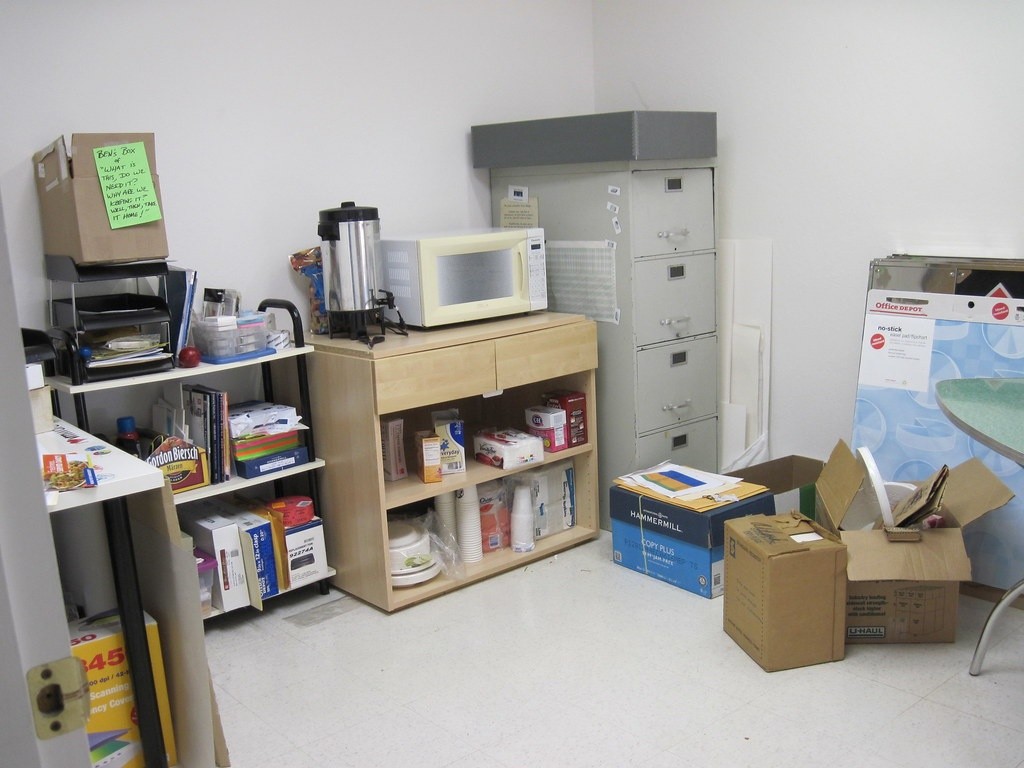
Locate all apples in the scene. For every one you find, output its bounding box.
[179,346,200,367]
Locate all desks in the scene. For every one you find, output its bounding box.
[935,376,1024,677]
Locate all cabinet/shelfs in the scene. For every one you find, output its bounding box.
[470,109,722,532]
[280,309,601,614]
[40,294,340,621]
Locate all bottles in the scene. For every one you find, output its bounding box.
[117,416,142,459]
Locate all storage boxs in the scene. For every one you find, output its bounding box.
[721,509,848,673]
[724,454,826,524]
[30,131,170,268]
[813,439,1016,644]
[609,467,775,600]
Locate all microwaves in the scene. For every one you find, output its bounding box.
[382,226,547,331]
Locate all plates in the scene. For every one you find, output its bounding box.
[50,460,88,489]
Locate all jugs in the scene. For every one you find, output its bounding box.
[317,200,395,340]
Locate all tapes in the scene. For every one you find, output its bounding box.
[266,329,291,350]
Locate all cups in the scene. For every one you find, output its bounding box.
[511,485,535,551]
[436,483,483,562]
